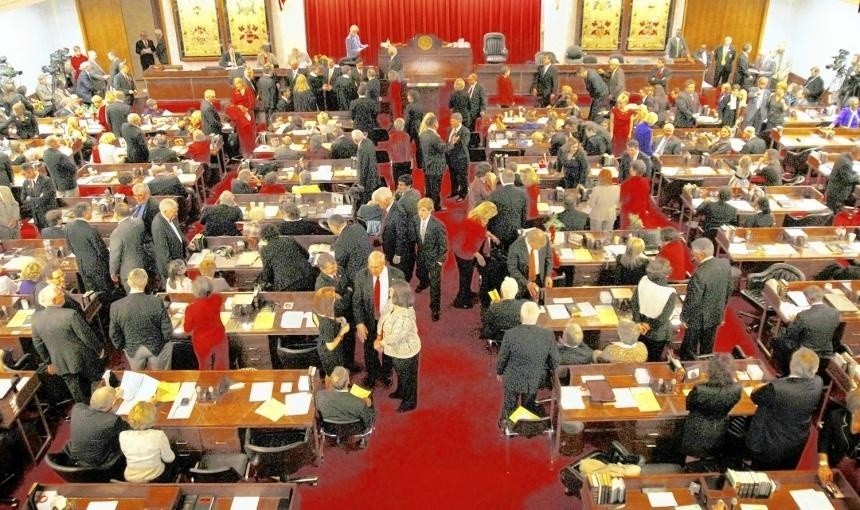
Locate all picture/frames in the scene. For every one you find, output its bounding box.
[575,0,626,56]
[170,0,227,62]
[219,0,276,61]
[623,0,676,56]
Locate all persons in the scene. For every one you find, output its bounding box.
[0,24,860,484]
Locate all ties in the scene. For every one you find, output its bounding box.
[528,249,536,281]
[372,276,380,321]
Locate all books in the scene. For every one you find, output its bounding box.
[586,473,626,506]
[725,468,776,498]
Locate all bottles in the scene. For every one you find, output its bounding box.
[655,377,679,395]
[194,384,219,403]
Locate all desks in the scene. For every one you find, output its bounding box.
[21,482,301,510]
[580,469,859,509]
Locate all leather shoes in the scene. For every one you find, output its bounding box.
[364,375,375,388]
[415,286,425,293]
[382,376,393,386]
[432,311,439,322]
[455,304,472,308]
[390,391,399,398]
[397,405,416,413]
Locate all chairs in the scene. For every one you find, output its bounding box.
[1,51,860,486]
[1,62,393,486]
[475,56,858,485]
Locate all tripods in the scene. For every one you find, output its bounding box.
[819,74,842,105]
